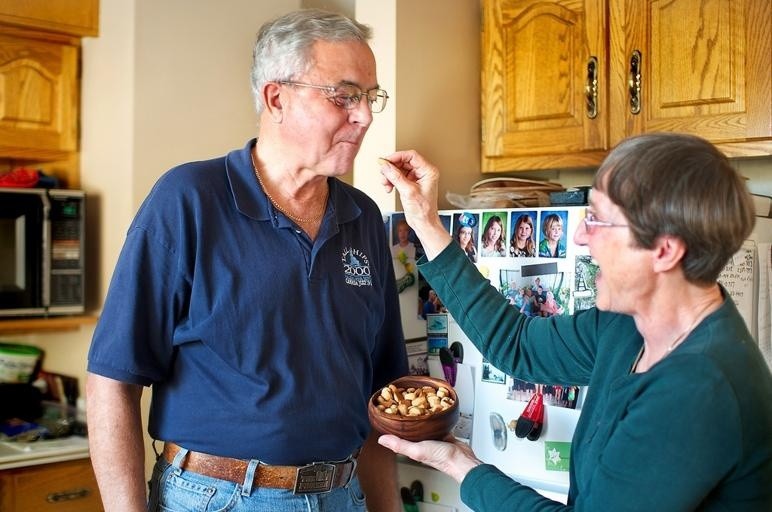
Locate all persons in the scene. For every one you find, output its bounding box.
[457,224,477,262]
[511,377,580,410]
[505,277,562,318]
[377,132,771,512]
[86,9,412,512]
[392,220,418,261]
[418,276,448,320]
[480,215,505,258]
[510,214,536,257]
[539,214,566,258]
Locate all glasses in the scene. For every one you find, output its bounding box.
[584,208,630,234]
[279,81,389,113]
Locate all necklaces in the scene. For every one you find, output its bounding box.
[250,153,329,222]
[630,295,721,374]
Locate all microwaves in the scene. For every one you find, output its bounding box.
[1,188,88,319]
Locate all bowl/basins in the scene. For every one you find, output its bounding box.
[367,376,460,442]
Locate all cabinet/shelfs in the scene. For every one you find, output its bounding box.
[0,32,81,152]
[1,457,105,510]
[477,2,771,172]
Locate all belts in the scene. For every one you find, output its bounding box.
[162,441,360,496]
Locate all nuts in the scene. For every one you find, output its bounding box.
[377,157,391,166]
[377,383,454,416]
